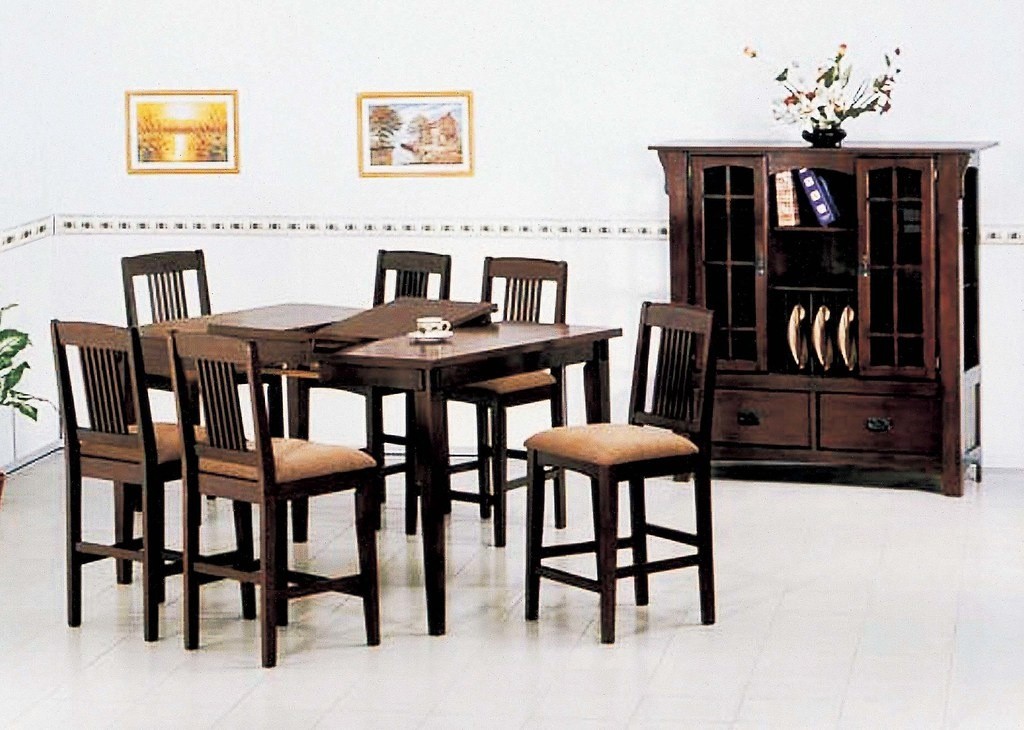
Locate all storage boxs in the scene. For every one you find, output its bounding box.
[775,171,802,227]
[798,169,839,225]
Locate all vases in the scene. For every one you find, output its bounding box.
[802,123,847,149]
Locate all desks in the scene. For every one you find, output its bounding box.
[127,301,624,636]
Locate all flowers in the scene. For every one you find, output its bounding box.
[742,42,901,134]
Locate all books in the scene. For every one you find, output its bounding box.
[774,168,840,227]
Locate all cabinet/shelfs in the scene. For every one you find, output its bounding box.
[646,140,998,498]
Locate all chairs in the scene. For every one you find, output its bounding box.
[307,247,451,528]
[166,328,381,667]
[122,249,283,501]
[525,302,716,644]
[50,320,255,643]
[406,258,569,547]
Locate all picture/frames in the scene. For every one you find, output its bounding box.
[357,92,474,179]
[125,91,240,175]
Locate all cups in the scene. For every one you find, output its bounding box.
[416,317,451,336]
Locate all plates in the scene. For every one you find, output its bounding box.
[407,331,453,343]
